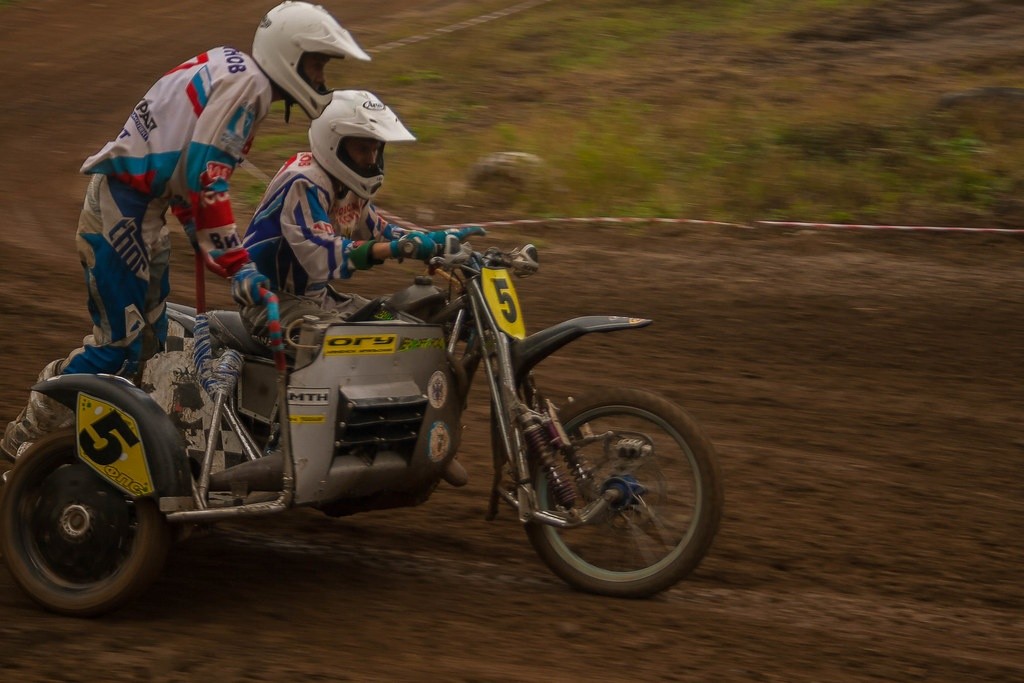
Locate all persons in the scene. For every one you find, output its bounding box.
[0,2,372,488]
[240,89,486,359]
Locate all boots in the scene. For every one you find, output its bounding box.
[0,358,79,465]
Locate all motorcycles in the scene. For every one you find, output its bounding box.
[1,236,724,614]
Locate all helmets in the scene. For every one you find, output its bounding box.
[304,88,417,200]
[252,0,372,122]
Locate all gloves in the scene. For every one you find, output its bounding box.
[424,224,487,259]
[230,262,271,309]
[391,231,438,265]
